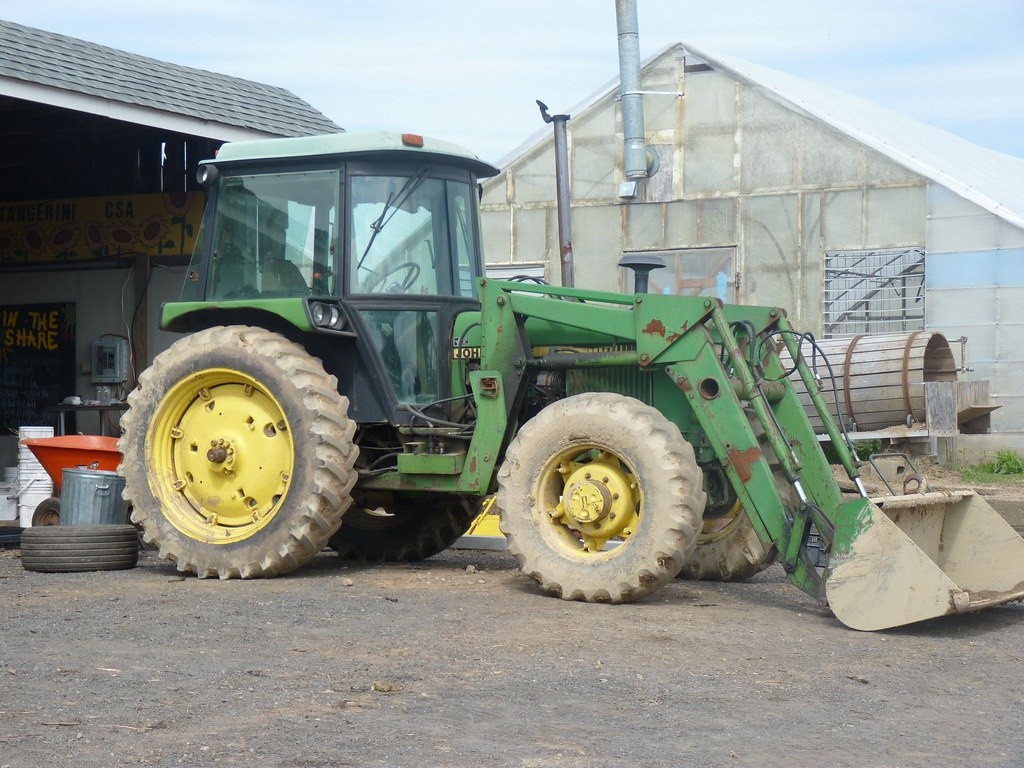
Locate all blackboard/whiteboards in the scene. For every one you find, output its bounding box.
[0,301,80,438]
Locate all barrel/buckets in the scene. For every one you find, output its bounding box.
[60,469,129,527]
[0,425,54,528]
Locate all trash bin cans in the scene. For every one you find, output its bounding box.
[61,467,130,528]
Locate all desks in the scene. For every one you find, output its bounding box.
[44,404,132,436]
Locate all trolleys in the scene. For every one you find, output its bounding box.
[20,433,123,528]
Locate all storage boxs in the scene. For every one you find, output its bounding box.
[0,494,20,520]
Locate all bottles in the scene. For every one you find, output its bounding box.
[96,386,112,406]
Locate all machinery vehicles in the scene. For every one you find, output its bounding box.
[115,1,1024,631]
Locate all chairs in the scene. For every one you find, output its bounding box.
[260,259,310,297]
